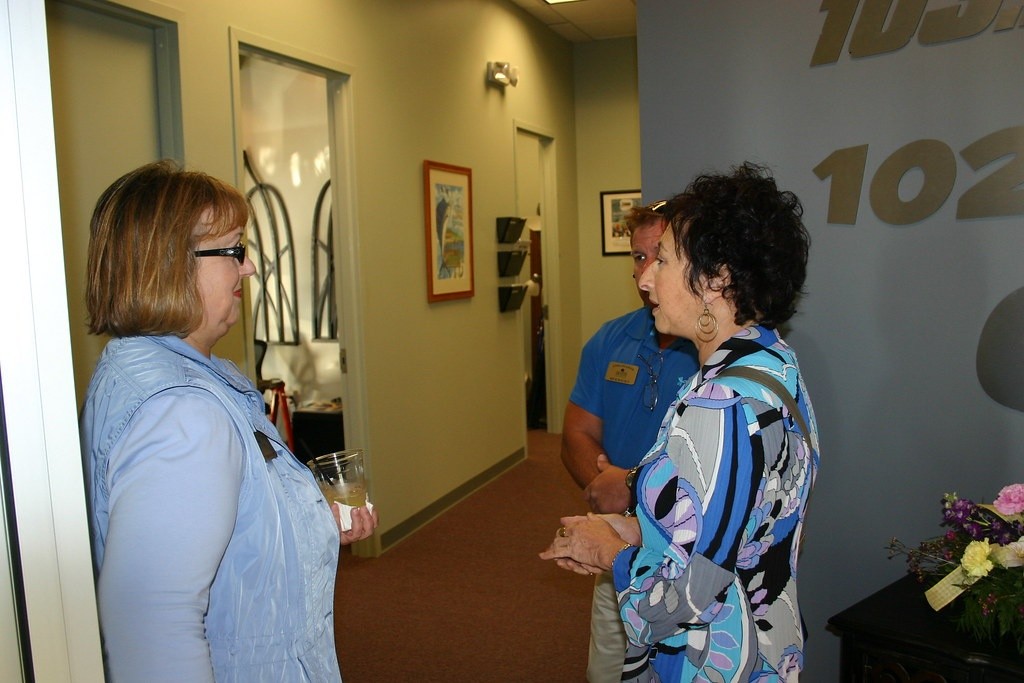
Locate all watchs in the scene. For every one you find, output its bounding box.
[625,466,638,491]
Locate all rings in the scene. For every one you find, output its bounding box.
[559,526,569,537]
[589,573,594,576]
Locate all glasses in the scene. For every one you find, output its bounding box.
[637,350,665,412]
[194,241,246,266]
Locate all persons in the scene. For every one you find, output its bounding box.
[77,158,380,683]
[537,160,822,683]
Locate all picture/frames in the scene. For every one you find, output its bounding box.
[600,189,643,256]
[422,160,474,301]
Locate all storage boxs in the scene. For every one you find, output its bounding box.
[497,250,527,277]
[497,217,526,244]
[498,285,528,313]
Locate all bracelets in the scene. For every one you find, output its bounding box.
[611,544,636,566]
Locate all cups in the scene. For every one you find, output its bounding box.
[307,449,366,510]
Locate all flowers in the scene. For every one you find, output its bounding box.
[884,483,1024,656]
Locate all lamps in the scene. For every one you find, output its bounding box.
[487,62,518,87]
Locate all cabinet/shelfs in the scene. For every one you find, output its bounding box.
[292,401,346,479]
[826,570,1024,683]
